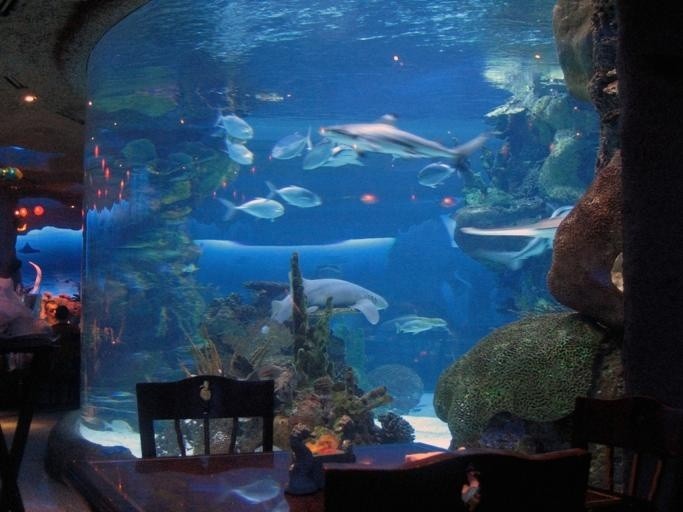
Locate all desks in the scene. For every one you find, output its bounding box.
[63,442,626,512]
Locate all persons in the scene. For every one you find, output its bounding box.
[51,306,80,369]
